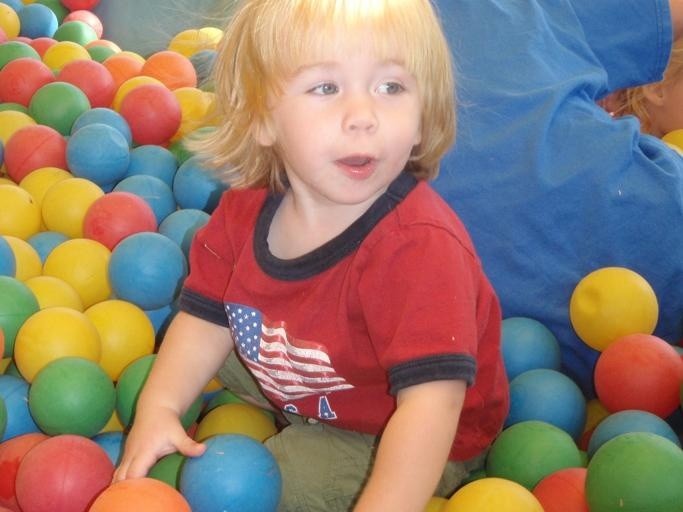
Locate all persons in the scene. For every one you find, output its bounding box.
[595,1,682,150]
[109,0,510,512]
[429,0,683,431]
